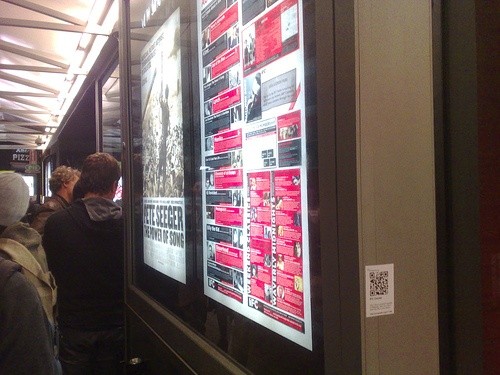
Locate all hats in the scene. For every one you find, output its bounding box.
[0,170,29,227]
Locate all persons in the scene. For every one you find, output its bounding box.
[0,165,80,375]
[154,84,170,199]
[44,153,126,375]
